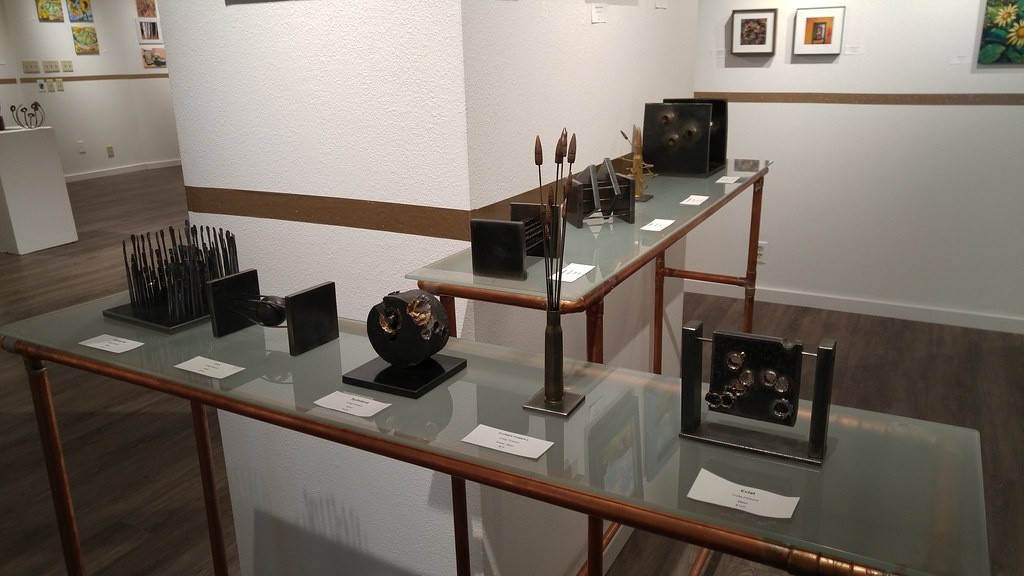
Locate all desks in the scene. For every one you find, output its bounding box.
[0,278,992,576]
[403,160,775,576]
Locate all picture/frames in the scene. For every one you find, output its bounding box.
[793,6,846,55]
[136,18,163,44]
[970,0,1024,73]
[731,8,778,54]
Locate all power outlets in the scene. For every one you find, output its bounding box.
[757,241,767,264]
[106,145,114,157]
[21,61,40,73]
[77,140,86,154]
[46,79,55,92]
[56,79,64,91]
[43,60,60,72]
[61,60,73,72]
